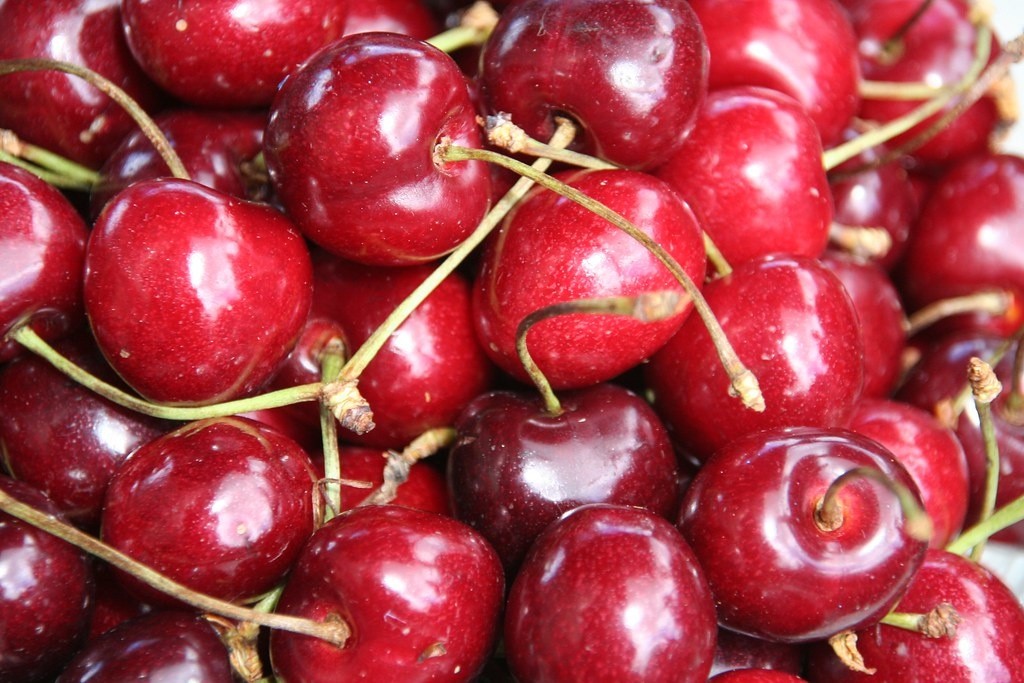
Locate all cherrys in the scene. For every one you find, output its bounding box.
[0,1,1024,683]
